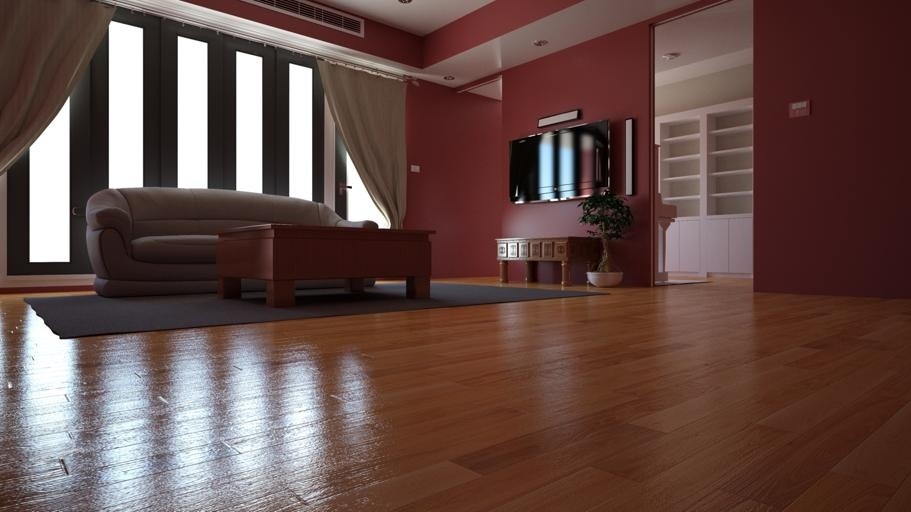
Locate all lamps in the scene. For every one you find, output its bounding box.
[538,109,580,128]
[625,119,635,196]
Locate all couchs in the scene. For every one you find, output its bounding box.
[85,187,378,298]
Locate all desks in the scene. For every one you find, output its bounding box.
[494,236,603,286]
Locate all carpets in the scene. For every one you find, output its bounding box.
[24,282,610,339]
[654,280,710,286]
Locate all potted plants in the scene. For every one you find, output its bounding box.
[576,187,636,288]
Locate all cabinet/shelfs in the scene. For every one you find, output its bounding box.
[655,97,753,278]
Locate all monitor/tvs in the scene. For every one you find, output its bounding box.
[509,120,610,205]
